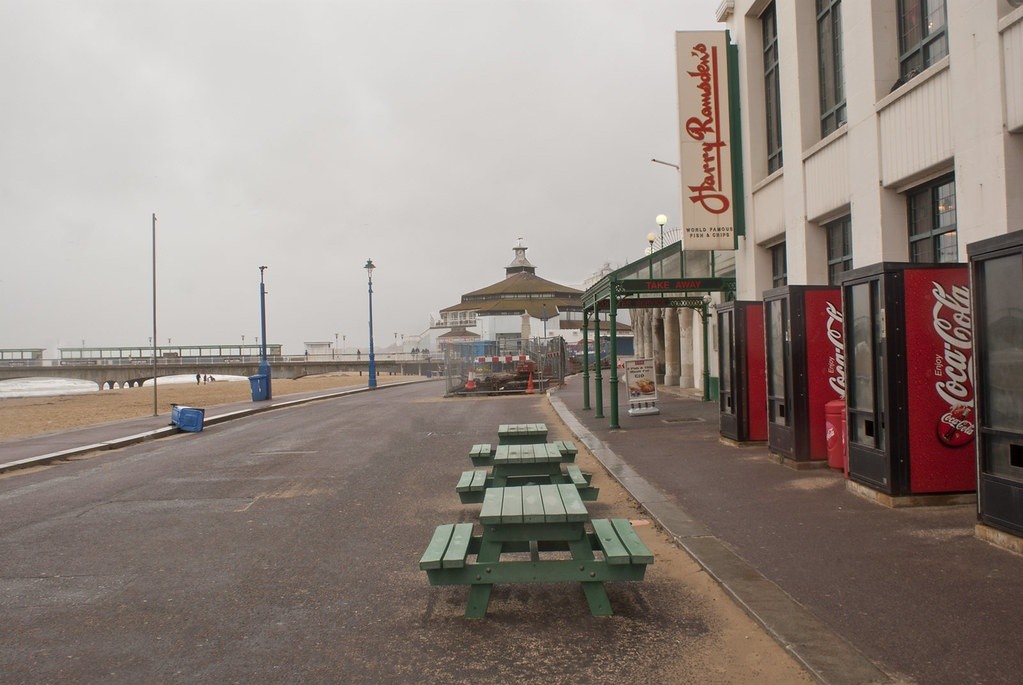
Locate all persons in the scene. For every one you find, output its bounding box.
[196,372,201,385]
[357,349,360,359]
[421,349,429,359]
[411,348,420,360]
[209,375,215,381]
[305,350,308,361]
[204,374,206,385]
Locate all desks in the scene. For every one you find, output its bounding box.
[463,423,614,620]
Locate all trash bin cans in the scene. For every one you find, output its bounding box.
[168,403,205,433]
[248,374,267,402]
[825,399,846,473]
[842,408,849,478]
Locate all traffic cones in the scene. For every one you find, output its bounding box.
[466,368,476,389]
[525,370,535,393]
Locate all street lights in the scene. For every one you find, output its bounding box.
[656,214,667,280]
[647,232,655,279]
[361,258,377,387]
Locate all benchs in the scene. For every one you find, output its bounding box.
[554,441,654,582]
[418,443,492,588]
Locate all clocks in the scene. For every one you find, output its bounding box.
[517,249,523,255]
